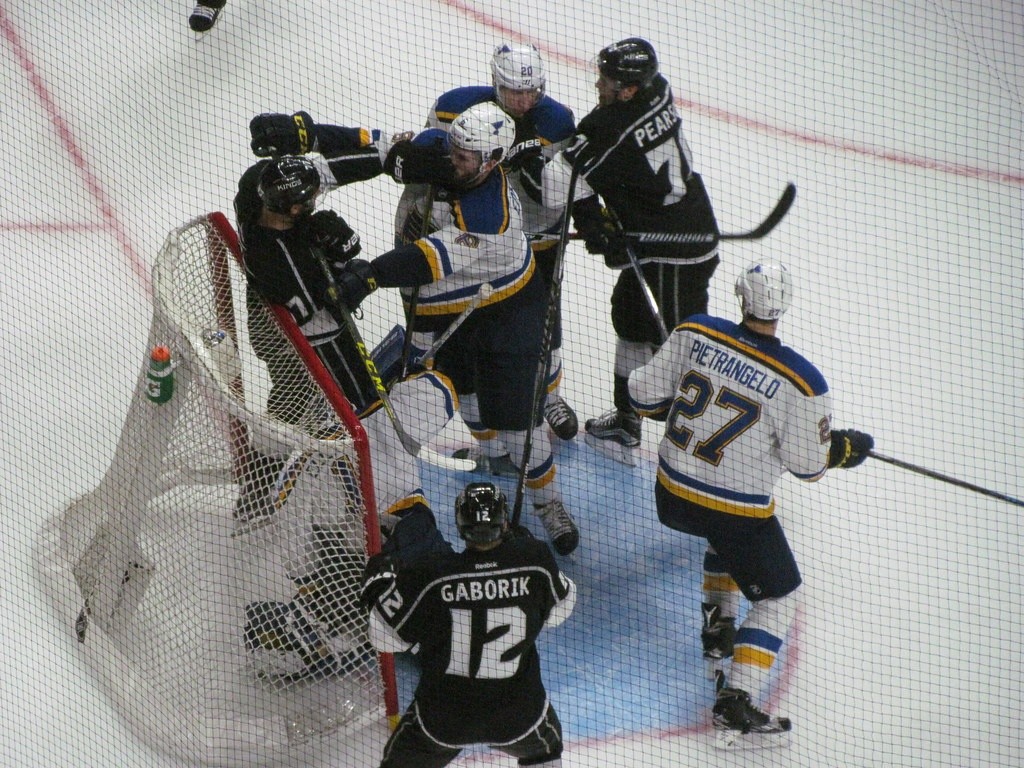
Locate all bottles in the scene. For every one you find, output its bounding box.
[143,346,174,404]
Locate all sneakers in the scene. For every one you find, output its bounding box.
[533,497,579,564]
[701,602,739,680]
[543,396,578,443]
[584,406,642,465]
[189,0,226,40]
[711,686,792,750]
[452,448,518,477]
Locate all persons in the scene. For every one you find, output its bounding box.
[395,42,601,447]
[251,101,580,563]
[630,256,875,750]
[189,0,227,42]
[516,38,722,465]
[233,140,378,518]
[244,370,458,653]
[366,483,577,768]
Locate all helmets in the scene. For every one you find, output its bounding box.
[454,482,508,542]
[735,259,793,319]
[257,154,320,214]
[489,41,545,90]
[598,37,658,86]
[448,101,515,165]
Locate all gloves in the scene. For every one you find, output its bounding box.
[828,428,874,470]
[249,110,316,157]
[362,553,399,599]
[325,260,375,313]
[584,223,615,255]
[382,139,457,185]
[309,209,362,263]
[573,194,604,255]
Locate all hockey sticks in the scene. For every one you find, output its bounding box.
[523,180,797,245]
[397,183,436,380]
[595,192,673,338]
[871,448,1023,508]
[511,165,579,528]
[413,294,480,365]
[312,242,479,474]
